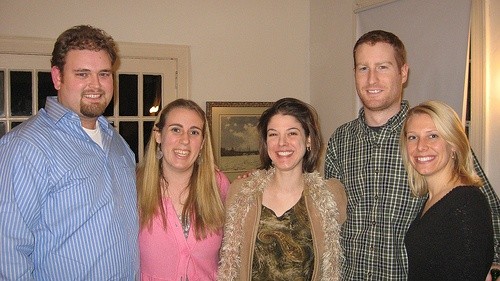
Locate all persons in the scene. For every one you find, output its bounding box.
[134,98,253,281]
[399,100,496,281]
[217,97,347,280]
[322,28,500,281]
[0,24,142,281]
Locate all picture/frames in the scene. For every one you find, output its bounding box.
[206,101,276,184]
